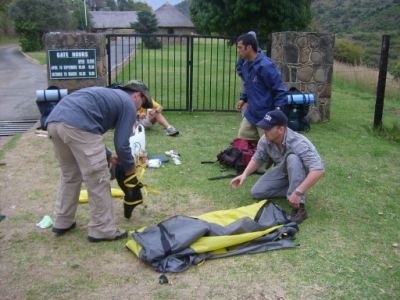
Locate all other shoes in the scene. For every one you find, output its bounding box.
[166,125,179,136]
[291,205,307,223]
[51,222,77,235]
[88,229,129,243]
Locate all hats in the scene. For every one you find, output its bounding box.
[255,110,289,130]
[114,79,154,109]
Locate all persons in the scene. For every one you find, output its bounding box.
[234,30,265,116]
[130,97,180,136]
[236,32,289,175]
[43,80,153,243]
[231,110,325,226]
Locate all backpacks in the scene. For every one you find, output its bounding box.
[286,87,311,133]
[201,137,258,181]
[35,85,68,130]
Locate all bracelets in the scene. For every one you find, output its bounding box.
[294,187,304,197]
